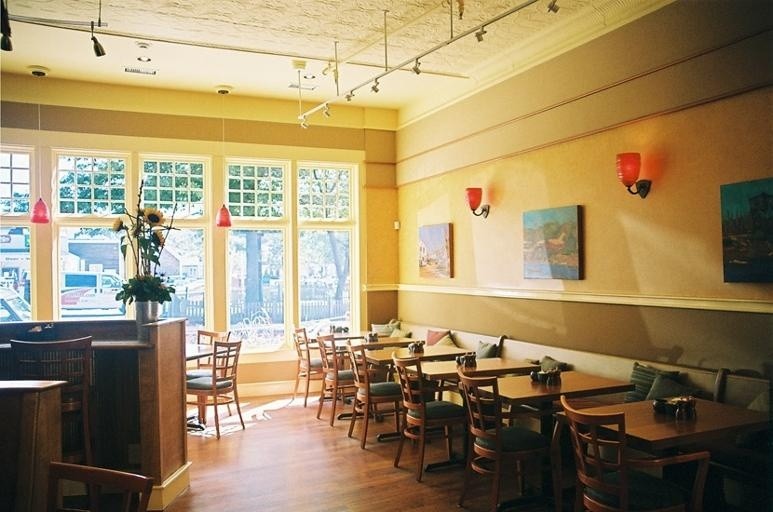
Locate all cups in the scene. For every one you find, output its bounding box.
[530,370,539,381]
[649,397,669,414]
[454,355,464,365]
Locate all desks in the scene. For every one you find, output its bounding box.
[313,329,391,339]
[355,344,476,366]
[184,339,234,360]
[330,338,426,351]
[404,357,543,406]
[476,368,637,438]
[555,396,770,512]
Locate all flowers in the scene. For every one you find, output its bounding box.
[104,175,186,302]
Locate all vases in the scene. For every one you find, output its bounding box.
[133,295,156,342]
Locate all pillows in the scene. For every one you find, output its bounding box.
[747,391,770,415]
[476,340,497,359]
[622,362,681,402]
[644,370,714,403]
[426,329,450,346]
[434,334,456,347]
[370,322,401,338]
[389,328,412,338]
[542,356,567,373]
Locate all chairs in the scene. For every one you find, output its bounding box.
[6,337,97,466]
[294,328,334,407]
[344,338,403,449]
[316,331,360,426]
[559,395,711,511]
[187,342,243,443]
[42,458,156,511]
[390,351,464,482]
[457,364,551,510]
[186,331,231,423]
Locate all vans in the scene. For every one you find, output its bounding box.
[61,272,126,314]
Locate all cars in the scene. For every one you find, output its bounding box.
[0,287,30,321]
[161,274,351,300]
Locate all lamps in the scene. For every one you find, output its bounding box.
[295,0,557,130]
[0,2,15,50]
[214,82,233,229]
[87,2,107,58]
[614,151,653,200]
[28,63,48,223]
[464,184,490,220]
